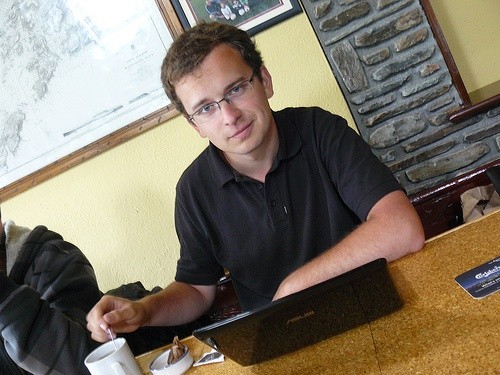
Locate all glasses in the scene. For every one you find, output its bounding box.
[187,71,255,123]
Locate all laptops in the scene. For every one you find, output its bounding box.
[193,258,403,366]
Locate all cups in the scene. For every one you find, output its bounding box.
[84,337,145,375]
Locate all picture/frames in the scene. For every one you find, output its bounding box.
[169,0,304,41]
[0,0,184,203]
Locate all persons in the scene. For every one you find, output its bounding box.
[203,0,251,21]
[0,209,101,375]
[84,23,427,345]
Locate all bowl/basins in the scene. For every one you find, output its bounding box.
[148,344,193,375]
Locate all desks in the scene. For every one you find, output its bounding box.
[132,211,499,375]
